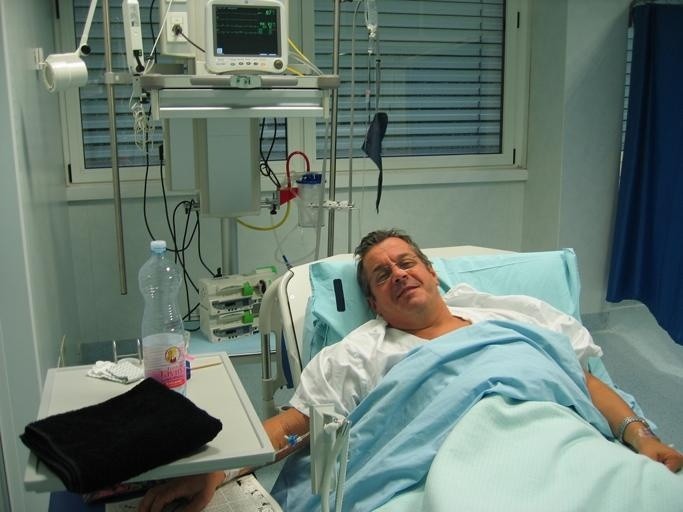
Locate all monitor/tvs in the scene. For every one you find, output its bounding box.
[209,0,287,75]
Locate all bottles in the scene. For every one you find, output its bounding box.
[137,238,187,399]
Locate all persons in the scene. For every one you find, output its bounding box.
[138,227,683,512]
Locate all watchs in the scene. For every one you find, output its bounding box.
[617,415,651,445]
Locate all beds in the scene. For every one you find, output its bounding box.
[258,244,683,512]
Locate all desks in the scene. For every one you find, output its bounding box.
[23,351,276,510]
